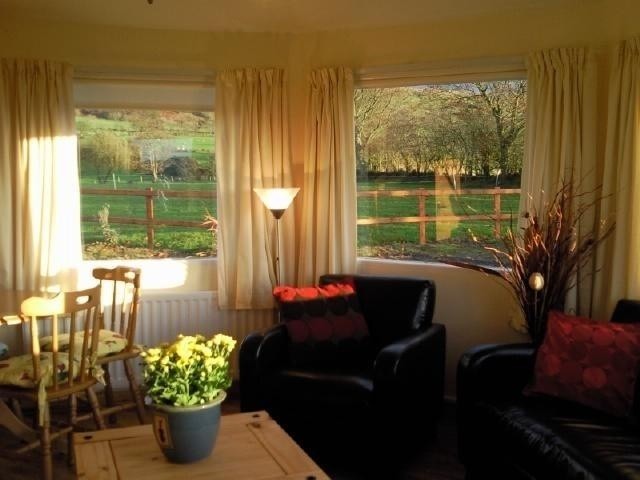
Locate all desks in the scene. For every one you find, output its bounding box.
[69,407,332,480]
[0,287,96,454]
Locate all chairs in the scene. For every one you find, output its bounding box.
[1,284,113,478]
[234,269,452,454]
[33,265,150,430]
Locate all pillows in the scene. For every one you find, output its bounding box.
[271,278,377,365]
[523,303,639,424]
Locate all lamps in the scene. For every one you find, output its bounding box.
[252,182,303,324]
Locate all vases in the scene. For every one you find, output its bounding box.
[137,387,228,465]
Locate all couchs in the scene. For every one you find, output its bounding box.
[451,296,639,479]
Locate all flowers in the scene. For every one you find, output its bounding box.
[129,327,245,411]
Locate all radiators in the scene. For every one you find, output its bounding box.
[19,287,280,396]
